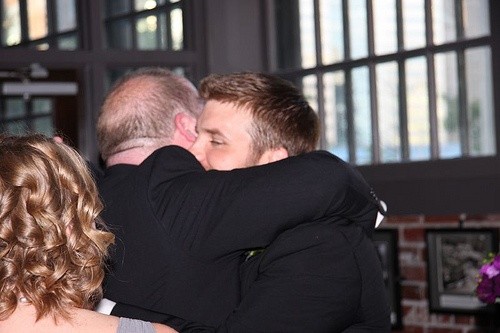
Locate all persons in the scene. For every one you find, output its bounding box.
[0,132,180,333]
[89,67,392,333]
